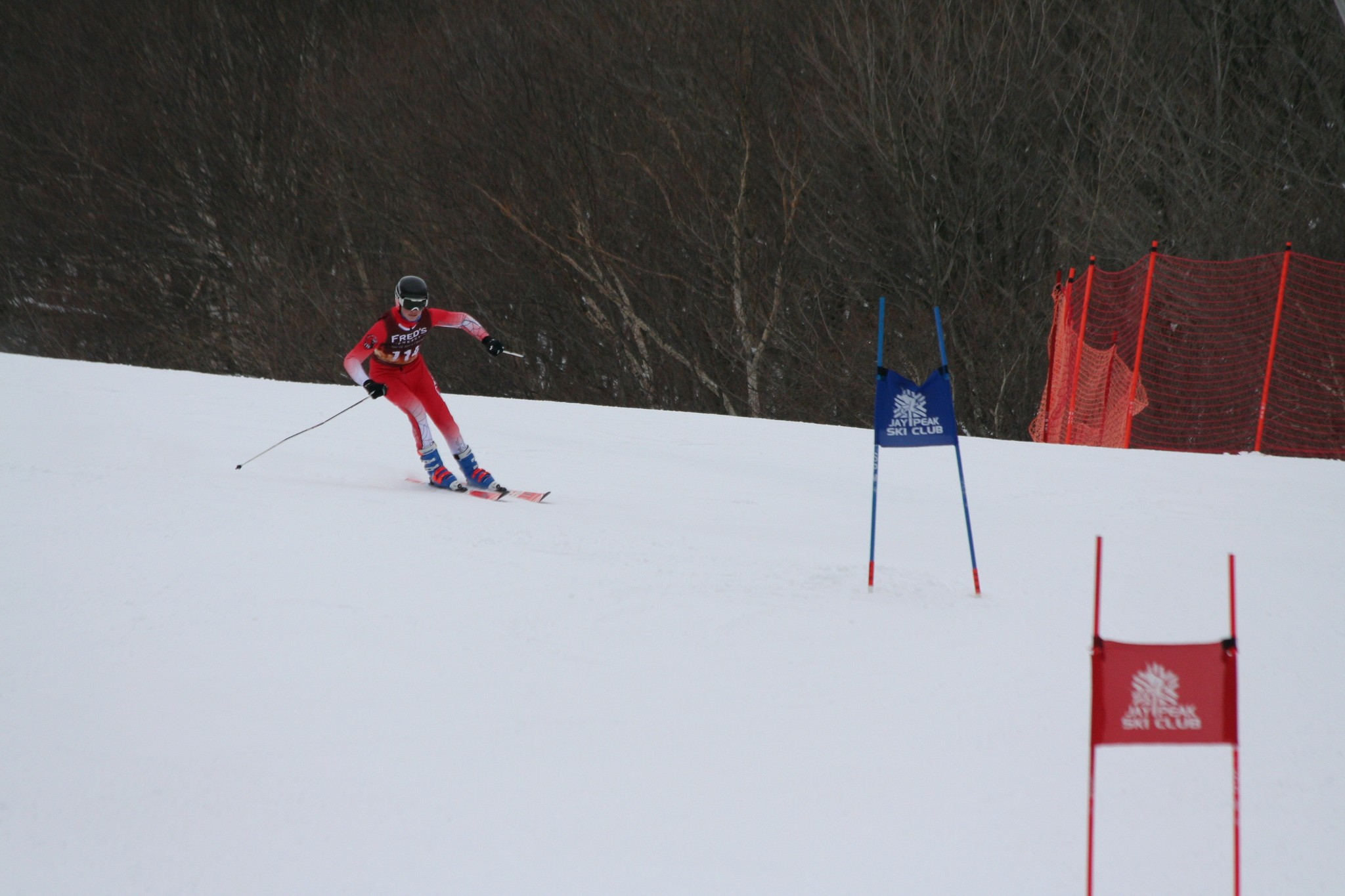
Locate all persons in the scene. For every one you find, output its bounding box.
[343,275,506,492]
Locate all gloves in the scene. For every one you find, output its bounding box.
[363,379,387,399]
[482,336,503,354]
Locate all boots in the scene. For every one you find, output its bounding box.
[454,445,498,489]
[417,442,459,488]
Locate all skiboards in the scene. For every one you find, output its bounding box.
[404,472,553,505]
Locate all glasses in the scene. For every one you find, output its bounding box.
[401,298,425,311]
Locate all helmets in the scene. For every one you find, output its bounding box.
[396,275,428,301]
